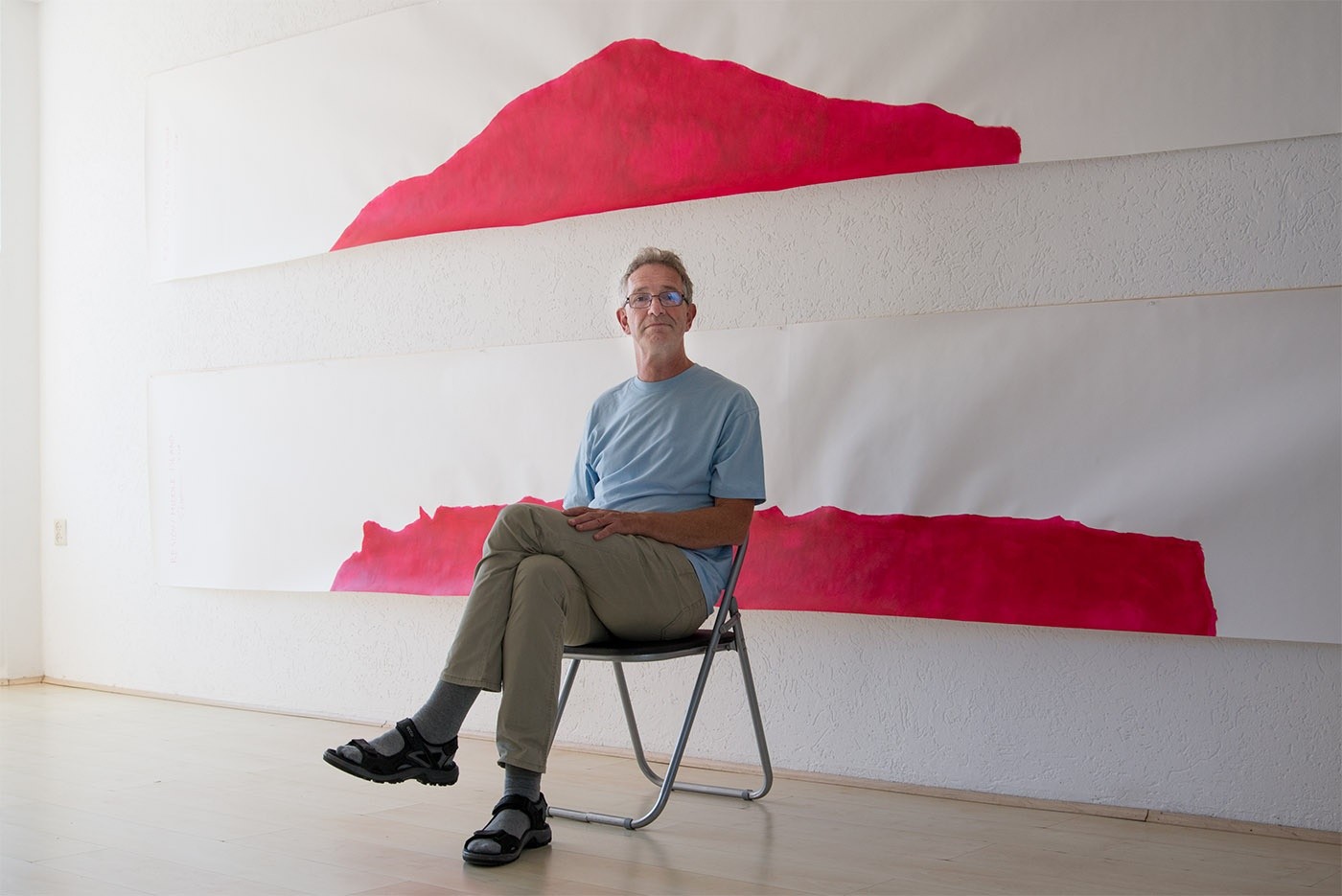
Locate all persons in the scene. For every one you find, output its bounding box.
[322,248,766,868]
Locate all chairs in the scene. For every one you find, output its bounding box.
[545,530,772,829]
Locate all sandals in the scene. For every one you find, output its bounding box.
[323,718,461,787]
[461,792,555,868]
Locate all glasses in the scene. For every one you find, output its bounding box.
[623,290,689,309]
